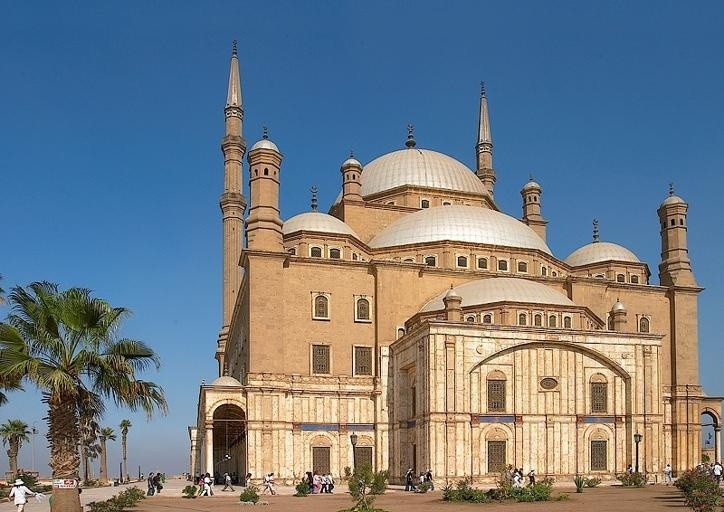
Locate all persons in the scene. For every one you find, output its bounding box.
[404,467,433,491]
[139,471,334,497]
[516,467,525,483]
[526,470,535,486]
[512,469,522,488]
[696,461,724,486]
[626,463,633,478]
[664,463,672,484]
[7,478,36,512]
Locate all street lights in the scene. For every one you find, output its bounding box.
[350,432,357,469]
[634,429,643,474]
